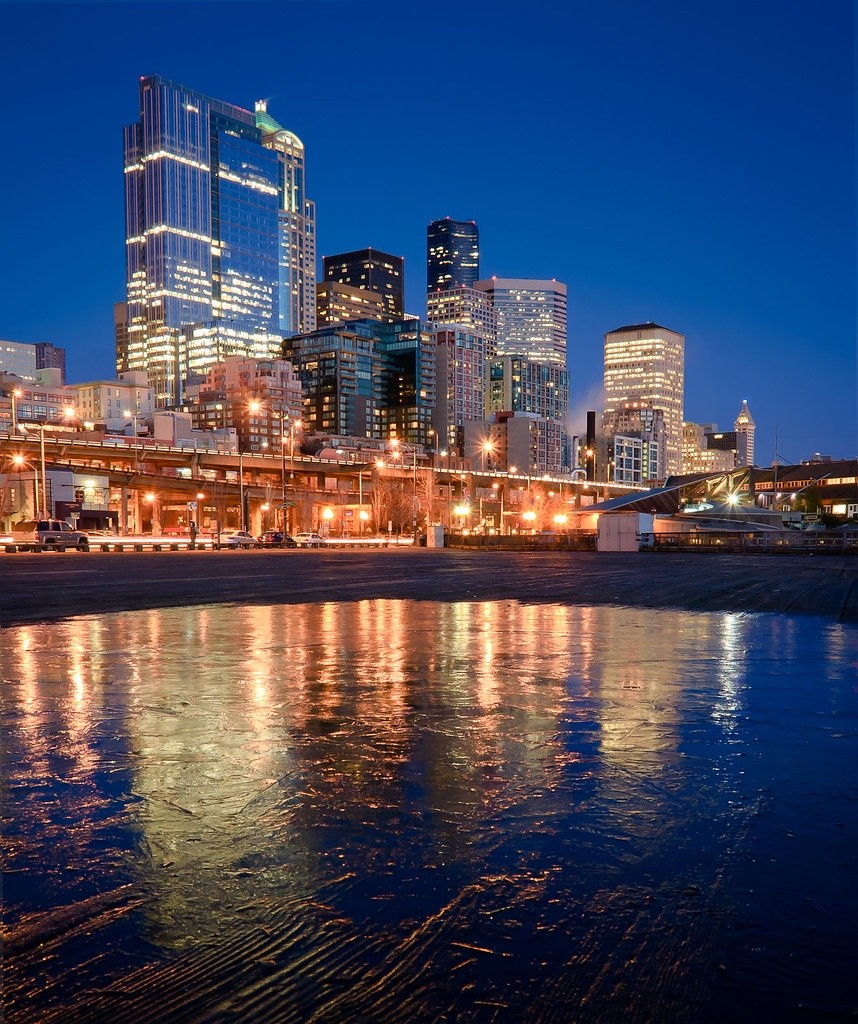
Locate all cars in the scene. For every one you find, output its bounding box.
[293,533,325,548]
[212,530,258,547]
[10,517,89,551]
[257,531,294,549]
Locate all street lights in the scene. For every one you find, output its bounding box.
[13,452,38,515]
[290,420,306,475]
[239,438,272,528]
[429,431,439,460]
[40,405,78,520]
[510,467,531,493]
[12,386,22,435]
[393,453,404,534]
[485,444,492,472]
[607,462,615,482]
[122,410,143,473]
[359,461,384,504]
[245,399,288,532]
[392,436,420,547]
[588,450,596,482]
